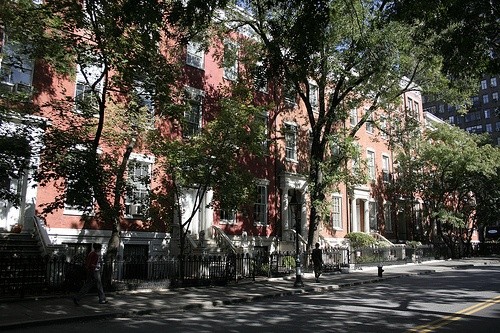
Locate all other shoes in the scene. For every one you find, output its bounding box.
[100,299,108,304]
[315,278,320,283]
[72,297,80,306]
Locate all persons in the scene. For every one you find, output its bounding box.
[73,243,108,306]
[312,243,324,280]
[432,232,469,260]
